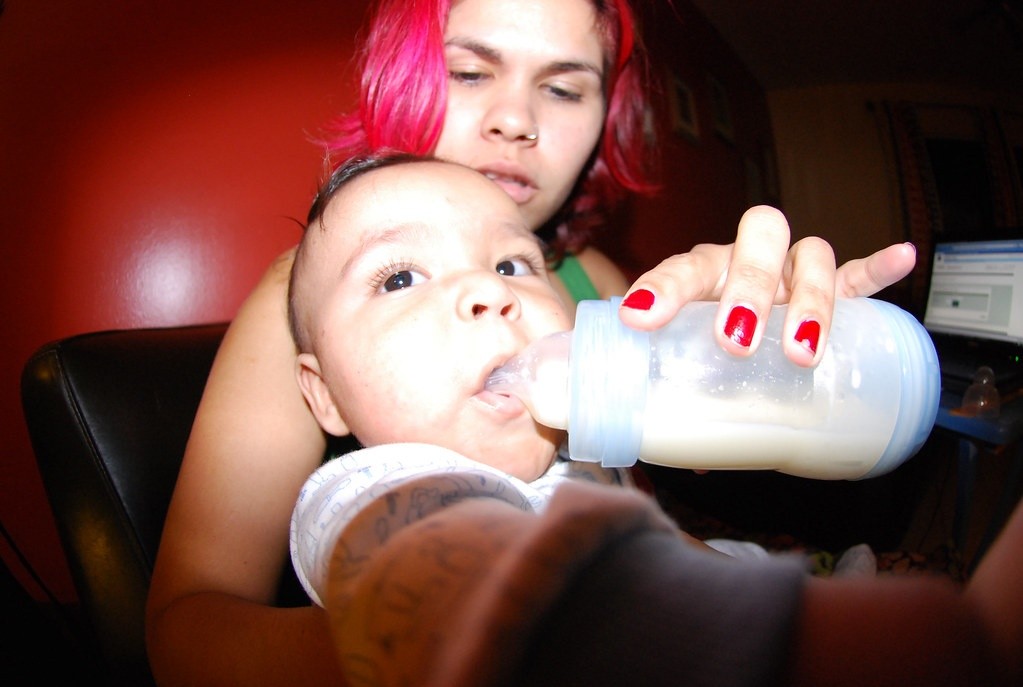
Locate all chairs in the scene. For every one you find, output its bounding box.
[21,322,364,687]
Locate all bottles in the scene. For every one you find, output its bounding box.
[486,294,944,483]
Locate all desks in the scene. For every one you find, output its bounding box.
[934,392,1023,554]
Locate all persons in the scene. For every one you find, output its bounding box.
[287,151,1023,687]
[144,0,918,687]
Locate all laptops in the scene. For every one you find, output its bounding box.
[920,236,1023,400]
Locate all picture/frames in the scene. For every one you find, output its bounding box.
[707,72,739,149]
[670,79,702,146]
